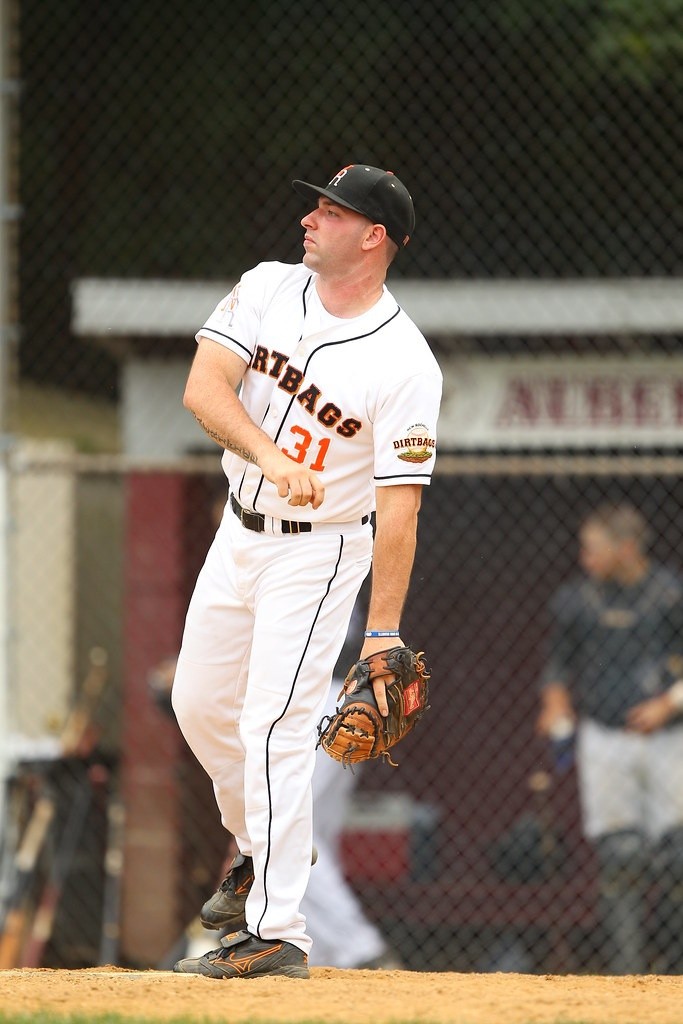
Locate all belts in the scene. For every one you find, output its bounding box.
[230,493,368,535]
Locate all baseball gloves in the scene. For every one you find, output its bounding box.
[315,644,432,766]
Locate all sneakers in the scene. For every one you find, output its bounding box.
[200,843,319,930]
[173,930,310,979]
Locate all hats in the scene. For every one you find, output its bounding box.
[291,164,415,250]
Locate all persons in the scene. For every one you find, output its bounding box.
[539,507,683,976]
[169,168,445,973]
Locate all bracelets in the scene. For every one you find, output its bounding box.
[362,629,402,638]
